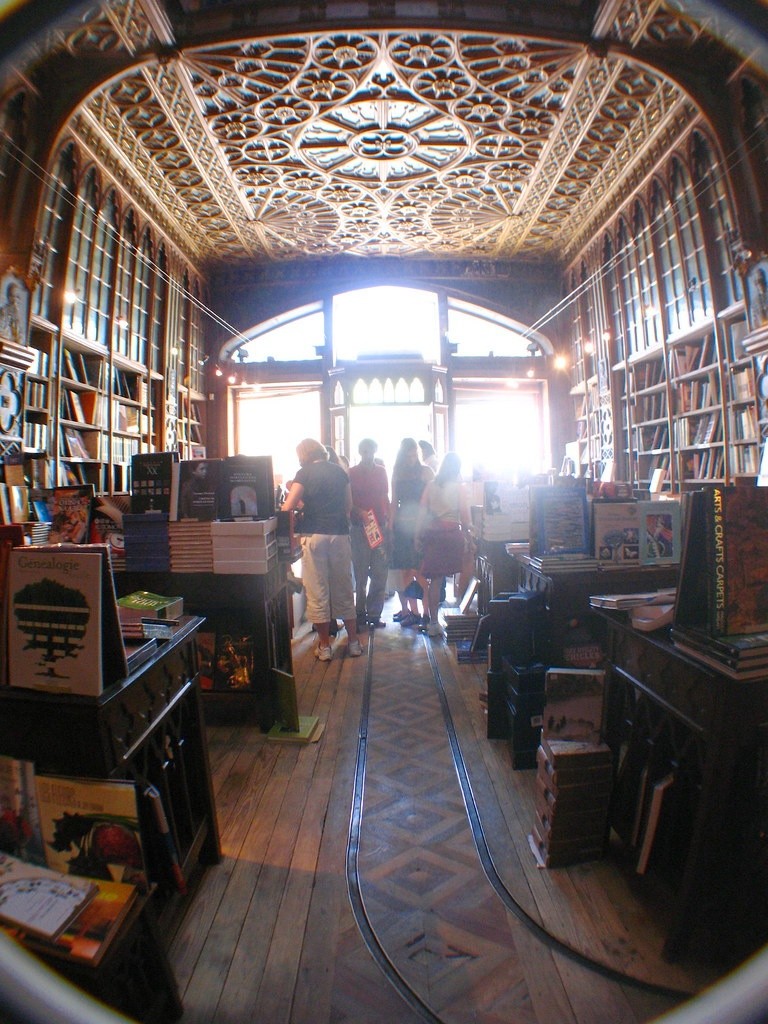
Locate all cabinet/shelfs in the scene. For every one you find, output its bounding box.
[0,303,768,973]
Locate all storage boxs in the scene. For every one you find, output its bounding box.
[545,667,606,745]
[537,746,616,785]
[539,729,613,767]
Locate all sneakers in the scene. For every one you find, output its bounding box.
[349,640,364,656]
[315,642,333,661]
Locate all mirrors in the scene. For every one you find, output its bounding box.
[0,0,768,1024]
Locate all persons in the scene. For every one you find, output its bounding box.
[279,438,364,660]
[752,267,768,329]
[347,438,394,634]
[286,437,441,632]
[0,283,24,341]
[413,452,477,636]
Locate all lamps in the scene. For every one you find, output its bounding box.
[215,366,225,377]
[313,344,324,359]
[228,373,237,384]
[527,342,539,357]
[448,343,457,355]
[237,349,248,362]
[197,355,208,365]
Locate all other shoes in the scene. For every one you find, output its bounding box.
[356,614,386,631]
[426,623,443,636]
[418,614,430,624]
[400,612,421,626]
[393,609,410,621]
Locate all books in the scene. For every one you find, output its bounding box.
[438,311,768,881]
[0,345,304,967]
